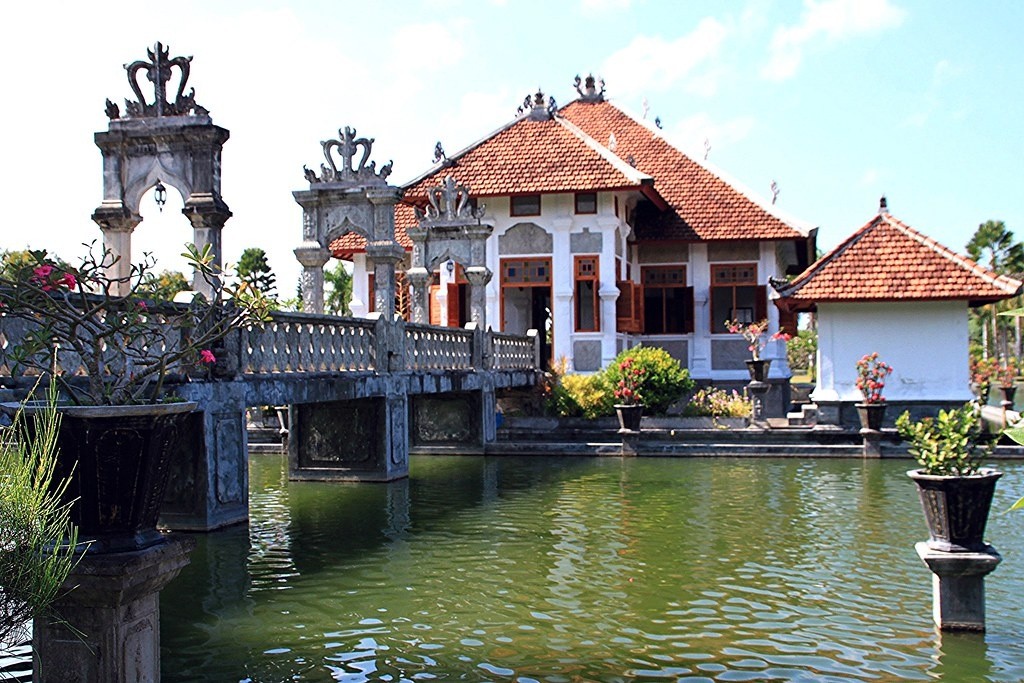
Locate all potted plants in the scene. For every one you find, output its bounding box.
[894,400,1005,553]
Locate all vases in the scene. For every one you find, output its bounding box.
[854,403,887,431]
[745,359,772,384]
[973,383,990,404]
[999,386,1017,410]
[789,369,815,400]
[614,404,644,431]
[1,400,198,557]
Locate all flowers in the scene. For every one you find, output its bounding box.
[0,239,273,405]
[613,357,645,406]
[854,352,893,404]
[972,354,1018,387]
[724,319,792,359]
[786,337,815,370]
